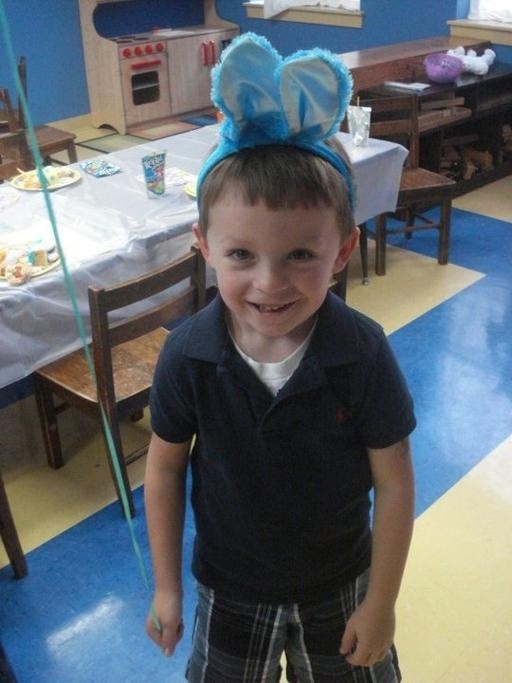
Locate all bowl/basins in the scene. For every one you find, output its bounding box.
[424,53,462,83]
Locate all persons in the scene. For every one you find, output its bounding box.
[143,111,418,683]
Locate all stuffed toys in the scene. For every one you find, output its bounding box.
[447,44,499,77]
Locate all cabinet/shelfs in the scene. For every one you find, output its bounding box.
[358,62,512,222]
[79,0,241,136]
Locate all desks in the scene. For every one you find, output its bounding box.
[339,36,491,93]
[0,124,410,389]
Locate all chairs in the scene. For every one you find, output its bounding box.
[0,56,78,184]
[0,476,29,578]
[33,243,206,518]
[352,93,457,276]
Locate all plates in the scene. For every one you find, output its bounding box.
[12,166,81,191]
[1,240,63,280]
